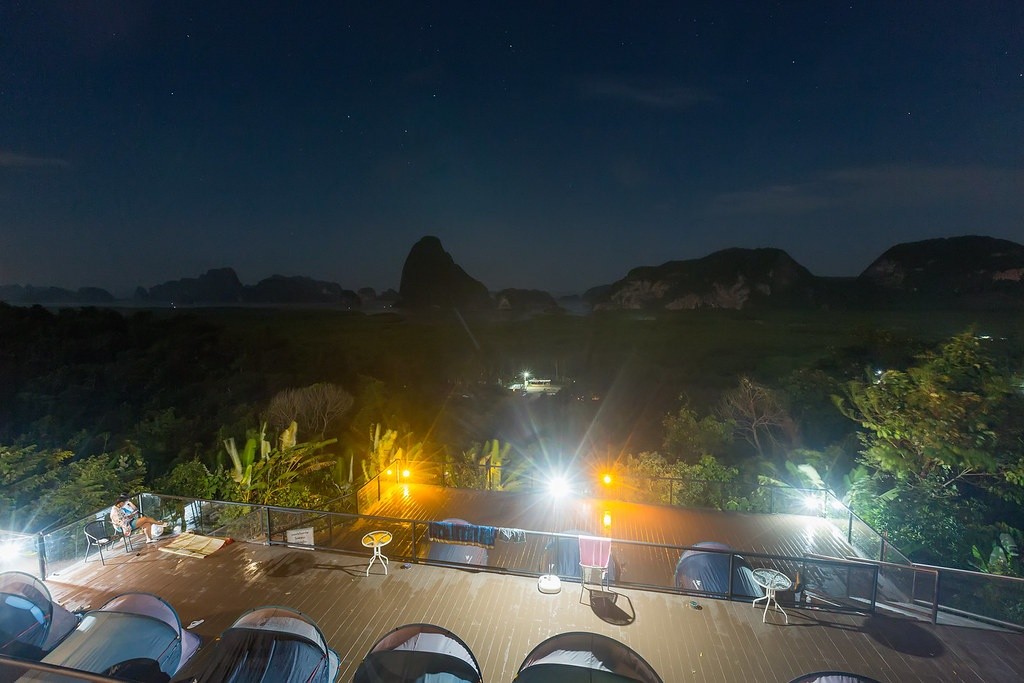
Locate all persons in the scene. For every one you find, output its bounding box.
[110,496,165,543]
[120,492,145,535]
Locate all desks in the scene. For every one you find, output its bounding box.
[751,568,792,623]
[361,530,392,577]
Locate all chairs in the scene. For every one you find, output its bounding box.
[83,520,128,566]
[107,512,147,552]
[578,535,616,611]
